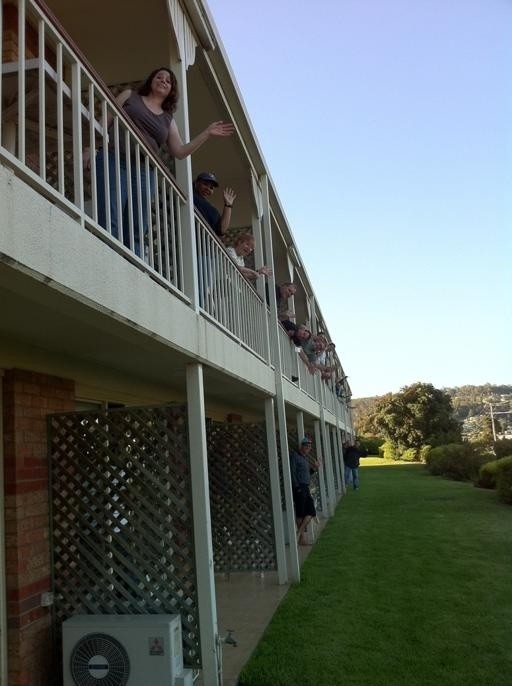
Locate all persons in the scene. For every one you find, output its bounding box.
[343,438,360,490]
[290,436,321,547]
[97,66,353,408]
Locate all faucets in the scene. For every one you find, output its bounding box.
[224,628,238,648]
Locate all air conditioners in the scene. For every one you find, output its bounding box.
[60,612,196,686]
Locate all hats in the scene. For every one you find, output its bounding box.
[198,172,219,187]
